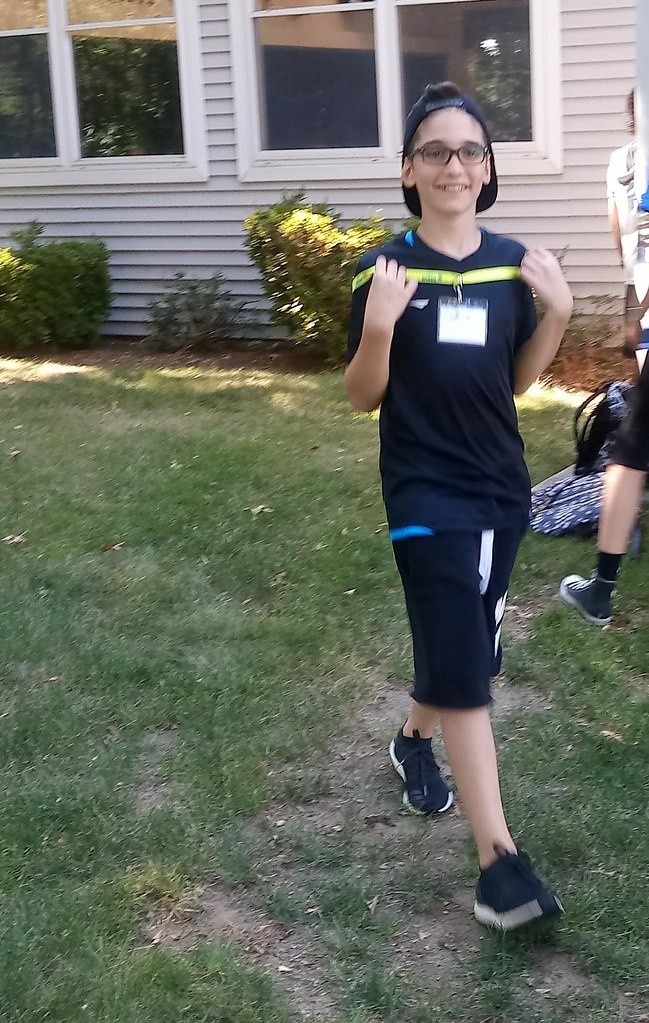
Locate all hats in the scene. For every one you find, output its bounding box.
[401,92,498,217]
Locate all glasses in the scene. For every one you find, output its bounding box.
[408,144,489,166]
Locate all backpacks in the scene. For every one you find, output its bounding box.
[572,381,633,475]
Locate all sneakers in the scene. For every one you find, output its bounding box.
[388,719,453,814]
[473,844,566,930]
[559,574,615,625]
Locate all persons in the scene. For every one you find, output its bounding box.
[343,80,573,932]
[561,89,649,622]
